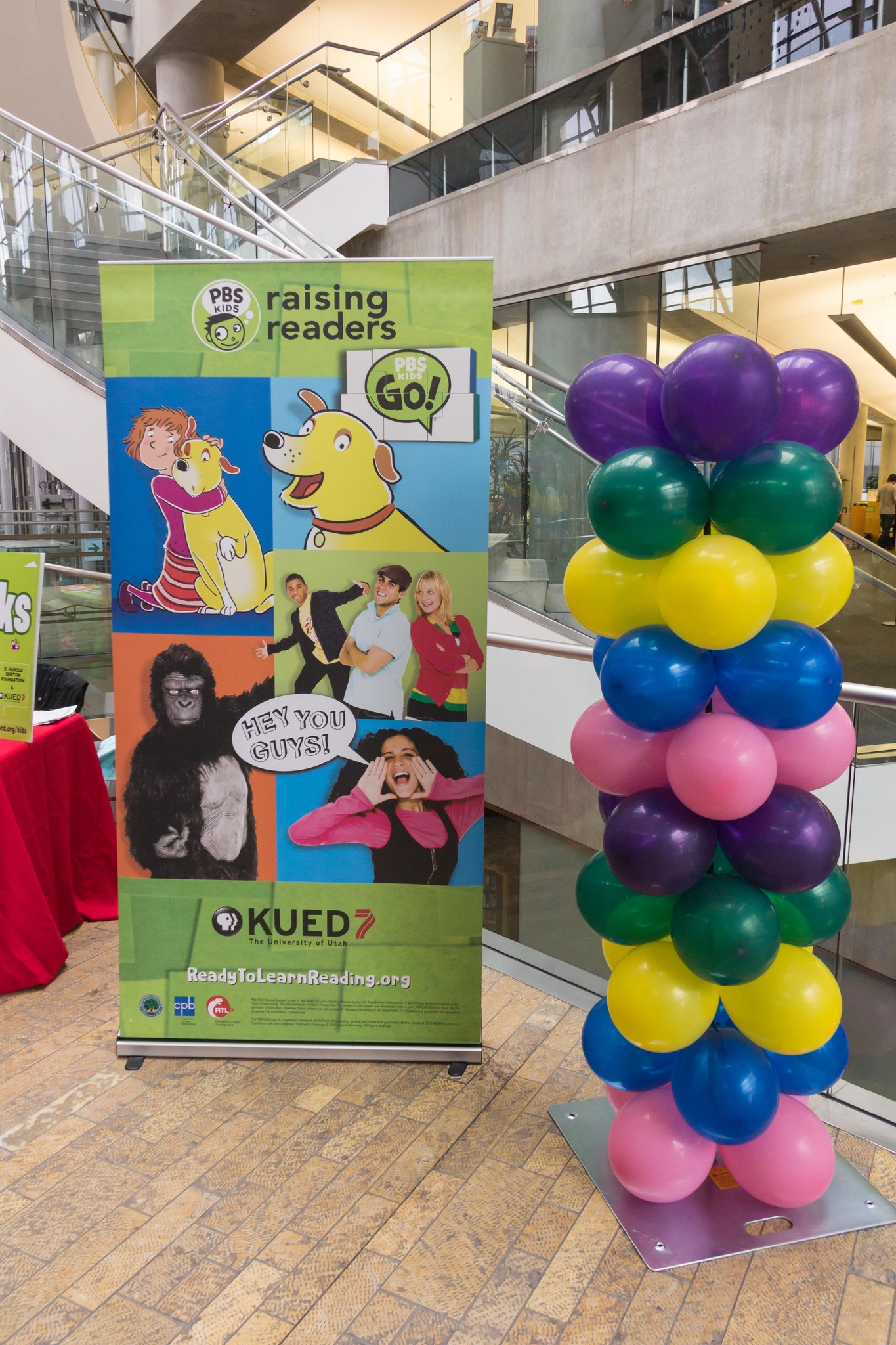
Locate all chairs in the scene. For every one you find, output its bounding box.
[33,662,90,715]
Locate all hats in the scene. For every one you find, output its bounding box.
[378,565,413,591]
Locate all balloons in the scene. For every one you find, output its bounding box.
[560,336,860,1208]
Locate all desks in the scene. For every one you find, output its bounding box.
[0,708,92,995]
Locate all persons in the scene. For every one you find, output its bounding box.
[255,563,484,885]
[877,474,896,550]
